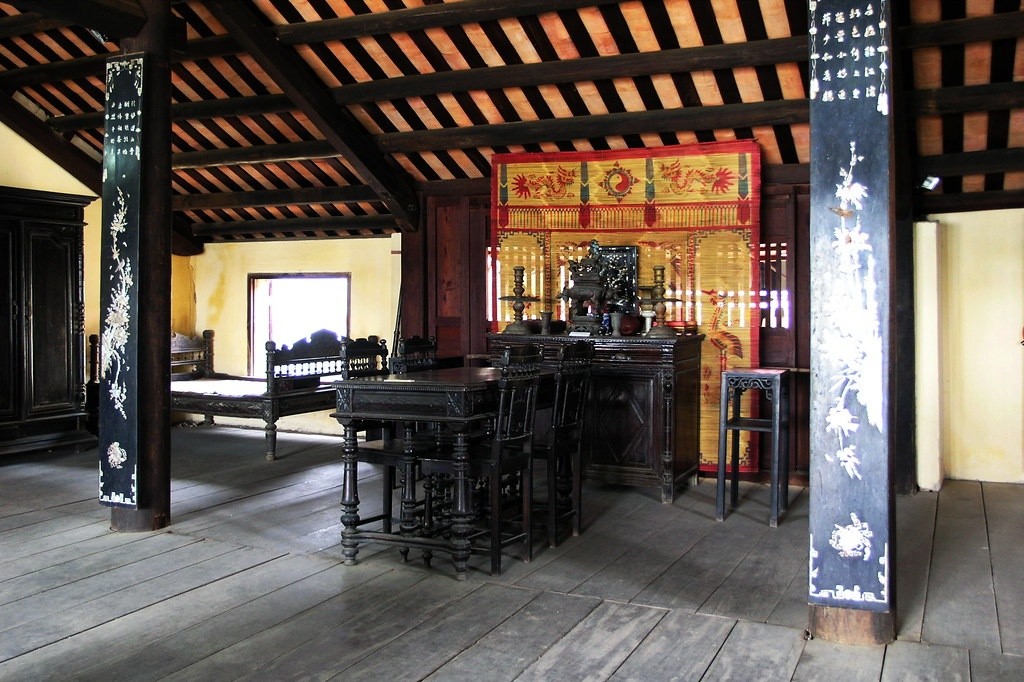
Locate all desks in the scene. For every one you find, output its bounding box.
[484,333,706,505]
[329,365,606,580]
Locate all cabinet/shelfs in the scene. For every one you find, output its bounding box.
[0,185,99,457]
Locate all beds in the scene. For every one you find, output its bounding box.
[85,330,378,462]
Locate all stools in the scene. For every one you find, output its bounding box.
[715,367,791,528]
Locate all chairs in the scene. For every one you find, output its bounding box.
[340,335,596,577]
